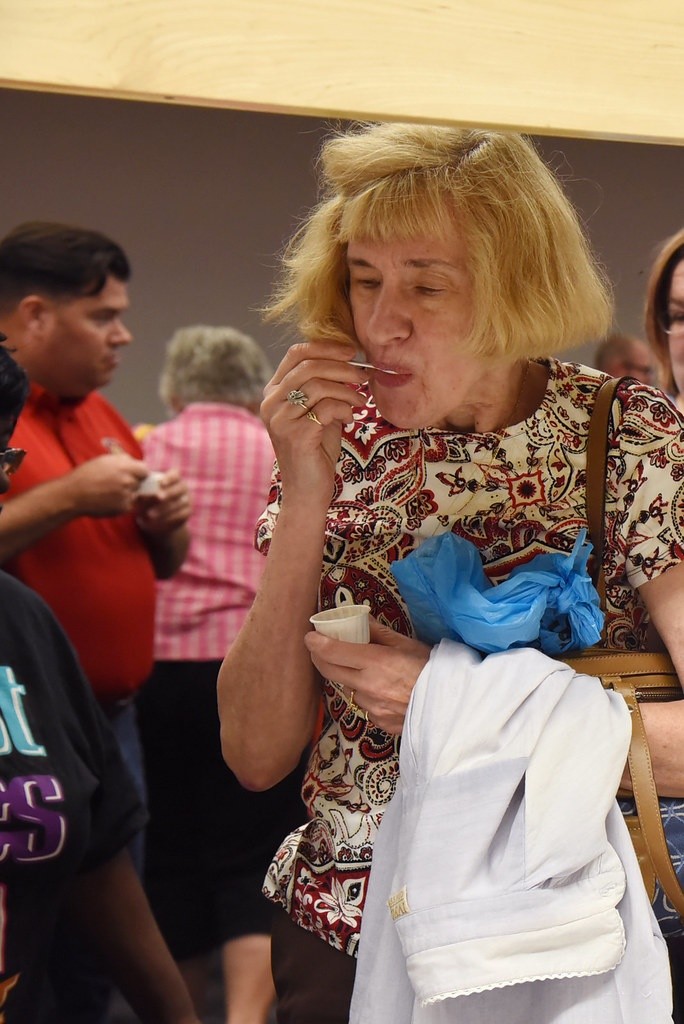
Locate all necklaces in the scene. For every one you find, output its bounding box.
[406,360,532,549]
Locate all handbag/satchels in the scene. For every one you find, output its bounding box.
[547,375,684,917]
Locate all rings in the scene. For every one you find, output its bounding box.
[364,710,369,722]
[287,390,311,411]
[349,691,359,714]
[306,411,324,426]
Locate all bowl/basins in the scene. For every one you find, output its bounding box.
[138,472,165,494]
[309,605,372,688]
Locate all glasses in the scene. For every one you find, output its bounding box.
[0,449,26,473]
[658,310,684,334]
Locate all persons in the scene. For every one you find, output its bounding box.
[0,222,294,1024]
[214,115,684,1024]
[592,225,684,417]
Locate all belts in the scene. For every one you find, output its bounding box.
[96,697,130,720]
